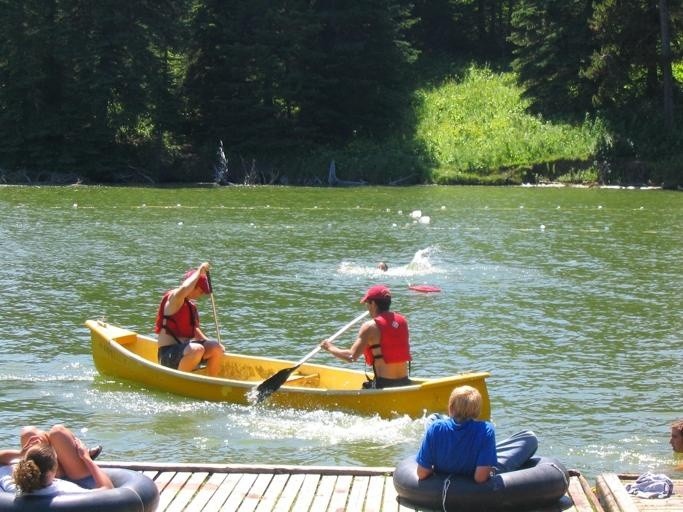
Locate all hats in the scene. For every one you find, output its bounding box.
[183,269,211,295]
[359,283,391,303]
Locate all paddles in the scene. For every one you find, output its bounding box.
[253,310,370,403]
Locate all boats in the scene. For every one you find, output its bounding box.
[82,315,496,431]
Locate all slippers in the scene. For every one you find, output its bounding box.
[87,444,103,460]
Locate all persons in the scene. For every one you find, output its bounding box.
[155,261,227,376]
[377,260,389,272]
[412,383,540,483]
[0,423,115,496]
[321,285,413,389]
[668,421,683,473]
